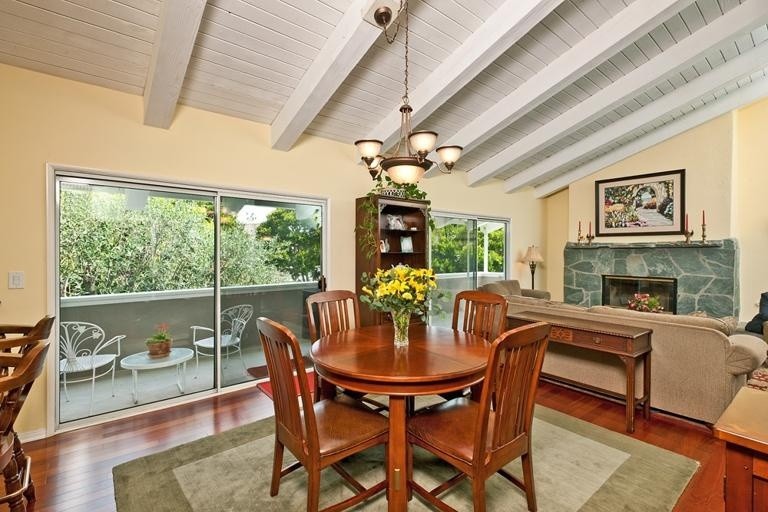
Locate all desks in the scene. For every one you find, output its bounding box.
[120,344,195,407]
[711,384,768,511]
[309,324,492,511]
[507,307,655,434]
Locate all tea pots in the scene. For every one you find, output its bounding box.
[392,263,409,269]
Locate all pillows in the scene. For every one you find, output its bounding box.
[687,308,706,318]
[715,313,740,334]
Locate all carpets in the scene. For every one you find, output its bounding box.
[107,393,703,512]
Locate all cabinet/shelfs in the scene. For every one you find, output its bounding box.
[354,195,432,328]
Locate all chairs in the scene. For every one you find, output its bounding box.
[188,300,254,380]
[0,337,53,512]
[741,293,768,334]
[475,277,552,300]
[436,289,509,412]
[256,315,389,511]
[0,313,59,504]
[405,320,553,511]
[306,291,360,417]
[60,319,132,405]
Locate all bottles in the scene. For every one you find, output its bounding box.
[380,237,390,253]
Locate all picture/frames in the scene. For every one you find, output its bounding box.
[592,168,688,238]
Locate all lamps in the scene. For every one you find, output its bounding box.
[521,243,545,290]
[352,1,463,192]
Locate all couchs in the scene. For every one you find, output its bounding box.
[492,294,768,434]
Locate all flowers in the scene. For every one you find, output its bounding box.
[360,263,454,341]
[627,293,666,314]
[144,320,171,344]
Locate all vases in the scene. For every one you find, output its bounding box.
[145,338,172,357]
[392,310,409,347]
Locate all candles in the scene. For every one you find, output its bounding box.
[588,220,592,236]
[684,212,689,232]
[578,220,581,231]
[702,209,706,226]
